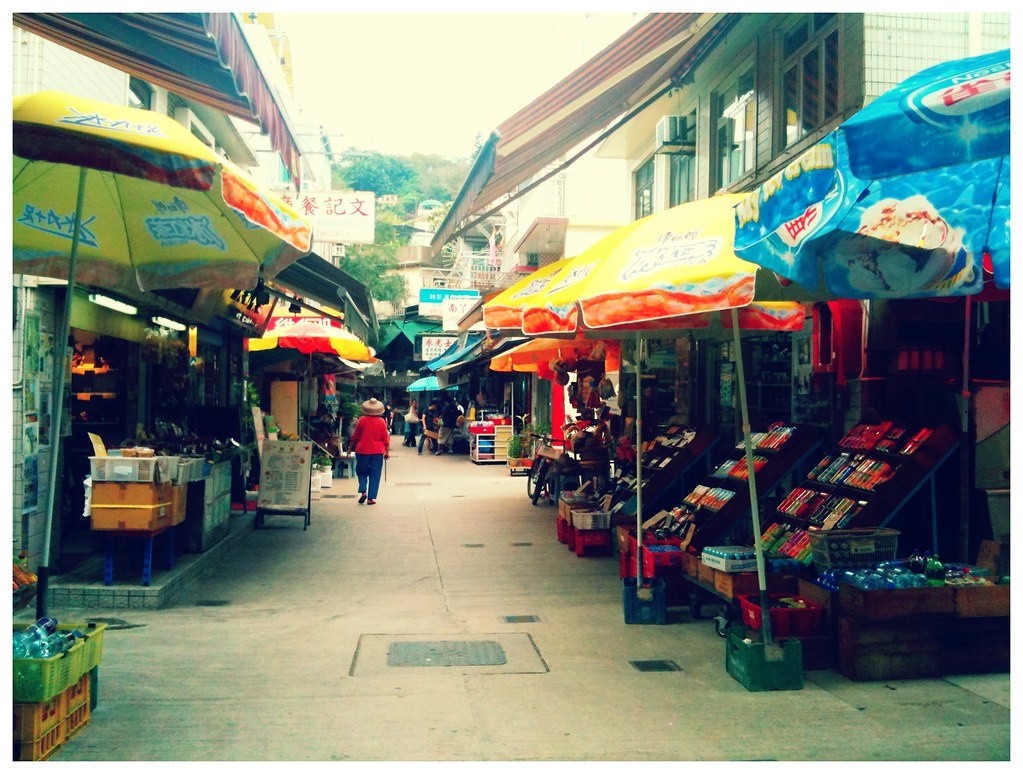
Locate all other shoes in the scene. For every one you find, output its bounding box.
[411,443,416,447]
[366,499,376,504]
[447,449,453,454]
[435,451,441,455]
[358,493,367,503]
[417,452,421,455]
[405,442,410,447]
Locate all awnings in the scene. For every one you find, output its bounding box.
[280,252,380,342]
[429,13,743,260]
[16,13,303,198]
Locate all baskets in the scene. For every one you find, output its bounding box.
[13,620,108,703]
[424,430,439,439]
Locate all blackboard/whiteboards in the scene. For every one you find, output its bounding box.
[256,439,313,514]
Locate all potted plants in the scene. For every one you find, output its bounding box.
[508,436,534,467]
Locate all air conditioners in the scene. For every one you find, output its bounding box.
[656,113,696,154]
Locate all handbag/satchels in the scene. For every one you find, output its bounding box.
[404,407,419,422]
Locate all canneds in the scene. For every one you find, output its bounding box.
[703,545,757,560]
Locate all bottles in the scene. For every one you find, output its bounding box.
[13,617,99,683]
[817,549,991,589]
[999,575,1009,583]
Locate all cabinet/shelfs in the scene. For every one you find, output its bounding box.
[469,422,514,462]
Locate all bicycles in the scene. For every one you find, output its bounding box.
[526,433,566,505]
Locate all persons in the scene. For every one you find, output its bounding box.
[417,397,464,455]
[315,410,359,451]
[383,403,405,435]
[402,399,418,447]
[621,381,653,444]
[347,398,390,505]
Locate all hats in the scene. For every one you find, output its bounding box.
[361,398,386,416]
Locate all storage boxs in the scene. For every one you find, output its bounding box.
[13,622,108,761]
[558,489,1009,693]
[88,454,207,531]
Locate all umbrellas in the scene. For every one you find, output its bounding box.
[406,373,459,400]
[735,51,1010,567]
[518,187,806,637]
[248,321,371,442]
[481,254,643,589]
[12,92,313,623]
